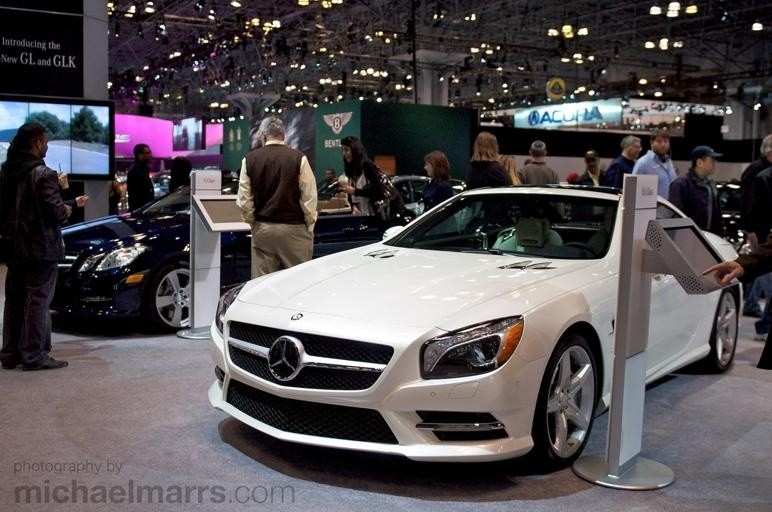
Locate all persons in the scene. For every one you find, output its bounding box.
[417,150,457,236]
[466,129,772,370]
[236,116,318,280]
[63,195,88,208]
[325,169,335,177]
[127,144,154,212]
[169,157,192,211]
[0,123,73,371]
[337,137,405,242]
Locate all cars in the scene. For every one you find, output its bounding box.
[713,181,746,246]
[316,176,466,219]
[153,173,171,187]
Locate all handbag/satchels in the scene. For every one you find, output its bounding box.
[364,167,404,224]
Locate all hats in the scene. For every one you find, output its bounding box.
[691,144,724,160]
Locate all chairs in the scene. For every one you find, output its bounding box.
[583,208,614,258]
[490,216,564,253]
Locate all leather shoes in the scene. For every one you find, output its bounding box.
[1,354,21,369]
[24,354,69,369]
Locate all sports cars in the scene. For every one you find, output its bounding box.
[50,181,412,336]
[205,184,746,471]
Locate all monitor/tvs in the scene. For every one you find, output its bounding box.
[172,115,207,151]
[191,193,251,234]
[645,216,737,294]
[0,93,115,184]
